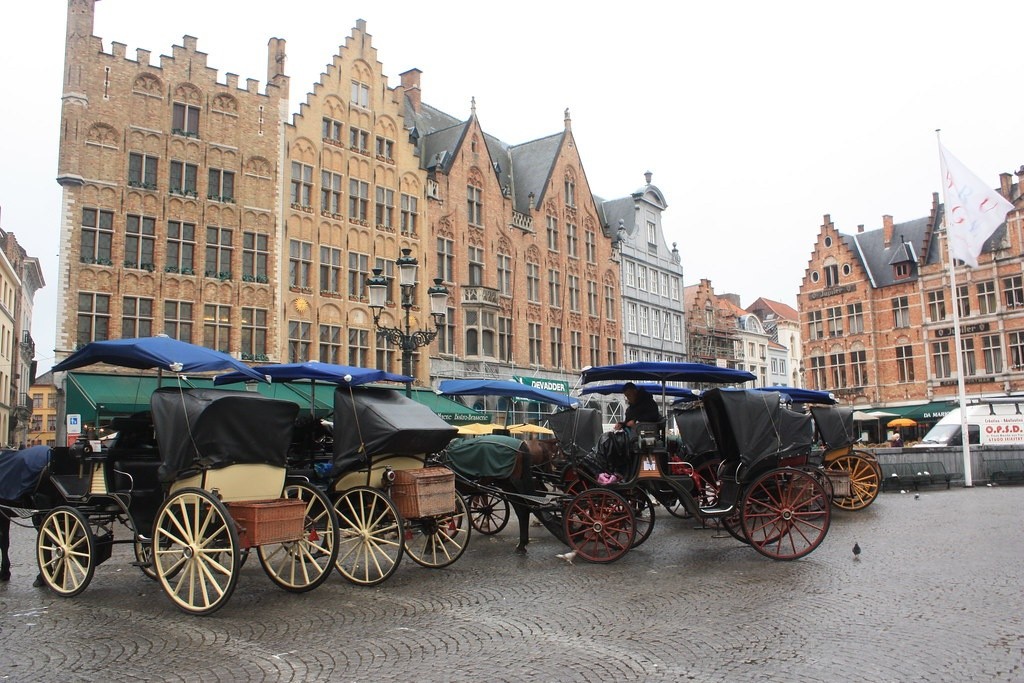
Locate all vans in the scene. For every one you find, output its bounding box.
[912,403,1024,446]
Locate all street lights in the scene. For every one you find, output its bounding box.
[366,247,449,401]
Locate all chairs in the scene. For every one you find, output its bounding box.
[85,418,153,495]
[631,416,667,454]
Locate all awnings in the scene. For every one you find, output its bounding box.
[65,371,491,427]
[856,406,925,419]
[901,403,960,420]
[887,418,917,428]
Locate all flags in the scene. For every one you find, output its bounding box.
[936,138,1015,272]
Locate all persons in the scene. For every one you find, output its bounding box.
[594,382,664,471]
[891,426,905,447]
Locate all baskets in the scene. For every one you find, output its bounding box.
[226,498,307,548]
[385,466,456,518]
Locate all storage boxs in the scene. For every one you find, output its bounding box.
[387,469,456,521]
[826,470,850,496]
[222,497,306,545]
[786,472,813,505]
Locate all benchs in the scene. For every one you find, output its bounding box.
[157,465,287,538]
[779,453,809,468]
[718,443,776,501]
[879,462,962,493]
[984,457,1024,484]
[823,444,850,460]
[333,454,426,490]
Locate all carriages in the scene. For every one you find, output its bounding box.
[0,336,883,617]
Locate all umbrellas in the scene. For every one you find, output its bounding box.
[437,379,580,435]
[748,385,837,410]
[51,335,271,391]
[577,383,701,397]
[853,411,879,445]
[582,361,757,448]
[867,412,900,445]
[213,360,414,417]
